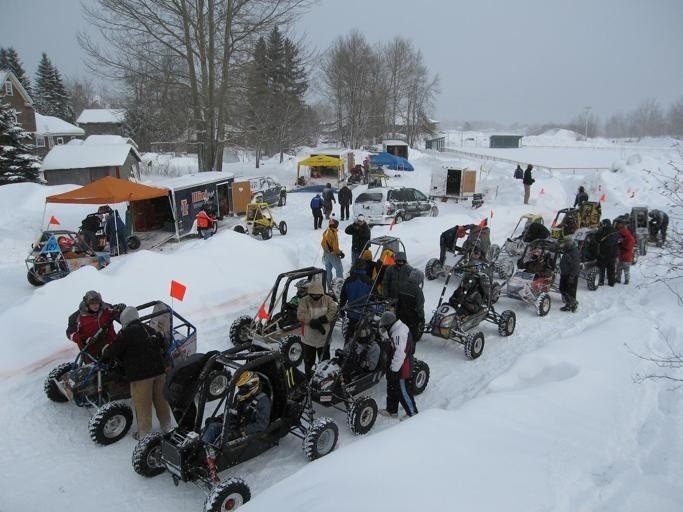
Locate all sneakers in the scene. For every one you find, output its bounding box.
[560,302,579,314]
[590,278,631,291]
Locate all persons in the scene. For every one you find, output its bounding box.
[104,212,127,256]
[101,306,173,441]
[82,213,102,249]
[248,206,262,221]
[196,209,213,238]
[202,371,272,445]
[163,349,224,426]
[66,290,126,366]
[378,312,418,419]
[58,236,91,258]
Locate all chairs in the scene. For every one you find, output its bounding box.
[254,370,275,408]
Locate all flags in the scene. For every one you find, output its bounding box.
[258,305,270,321]
[50,216,61,226]
[170,280,187,302]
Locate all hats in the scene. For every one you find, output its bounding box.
[357,216,364,222]
[83,291,103,306]
[327,218,339,228]
[118,306,141,330]
[379,311,399,328]
[294,278,311,290]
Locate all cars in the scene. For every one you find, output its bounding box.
[237,173,290,209]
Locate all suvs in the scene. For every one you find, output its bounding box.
[352,185,440,227]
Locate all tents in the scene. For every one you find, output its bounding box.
[370,151,412,170]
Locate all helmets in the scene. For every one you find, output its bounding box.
[354,319,373,344]
[235,370,260,403]
